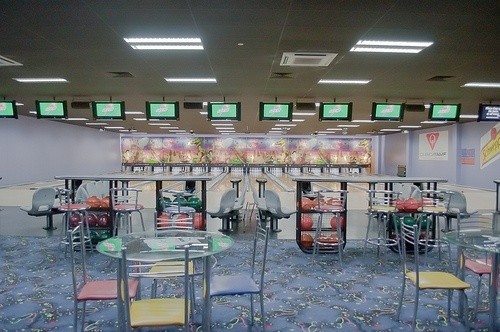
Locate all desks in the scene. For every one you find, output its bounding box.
[291,176,448,255]
[441,230,500,332]
[95,228,235,332]
[54,174,212,231]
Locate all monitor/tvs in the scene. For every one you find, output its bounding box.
[146,101,180,120]
[477,104,500,122]
[35,100,68,119]
[371,102,405,122]
[319,102,353,122]
[0,100,18,119]
[208,102,241,121]
[92,101,126,120]
[428,103,461,121]
[259,102,293,121]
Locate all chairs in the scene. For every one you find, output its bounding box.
[19,184,500,332]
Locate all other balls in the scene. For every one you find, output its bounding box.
[176,213,189,229]
[330,217,344,229]
[66,195,110,238]
[301,234,314,248]
[328,199,342,210]
[158,196,171,208]
[158,215,171,230]
[315,233,340,243]
[173,197,187,207]
[193,212,203,230]
[300,216,314,229]
[314,198,327,211]
[301,196,314,210]
[187,198,202,209]
[392,198,432,230]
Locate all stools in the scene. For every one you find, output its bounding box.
[109,188,146,237]
[416,190,455,268]
[158,189,195,231]
[56,187,94,258]
[364,189,402,270]
[312,188,349,265]
